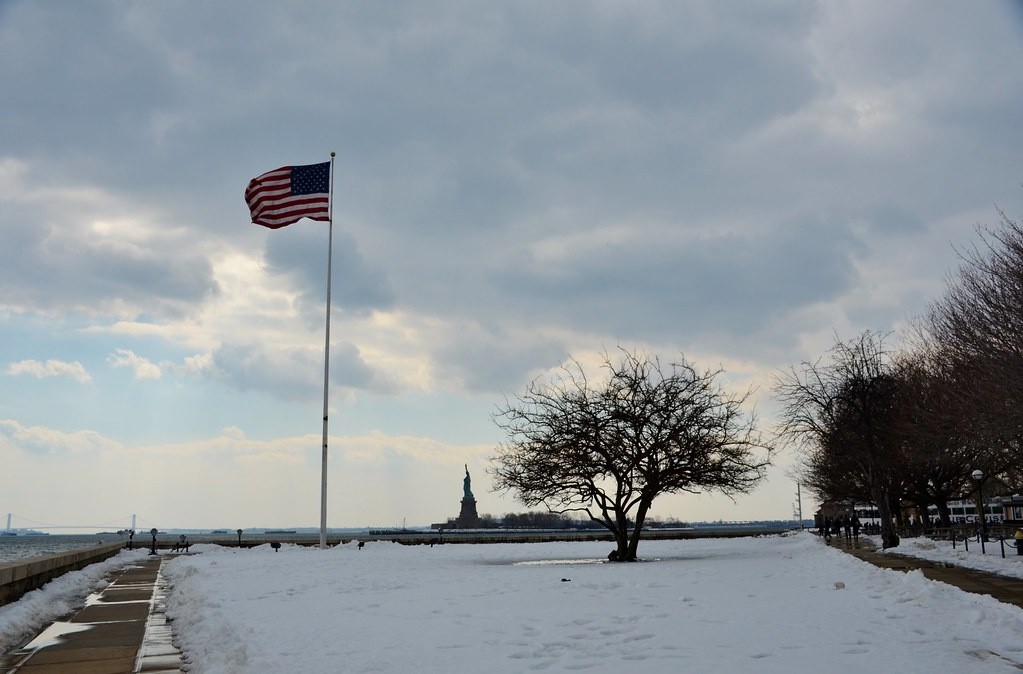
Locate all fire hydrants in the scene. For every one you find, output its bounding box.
[1013,530,1023,555]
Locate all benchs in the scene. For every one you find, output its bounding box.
[171,541,193,553]
[866,523,1011,540]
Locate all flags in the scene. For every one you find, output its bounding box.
[245,161,333,229]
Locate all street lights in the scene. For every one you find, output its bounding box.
[971,469,989,542]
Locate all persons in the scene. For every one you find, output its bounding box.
[823,511,863,540]
[863,516,943,538]
